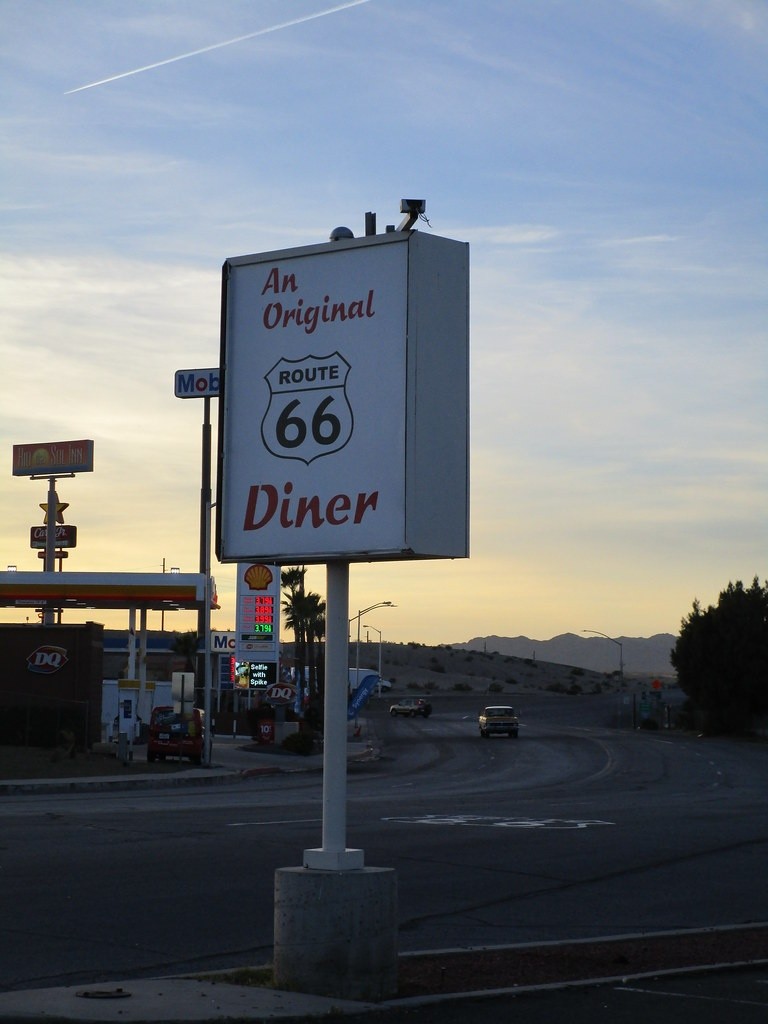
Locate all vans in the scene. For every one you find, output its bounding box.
[147,706,216,764]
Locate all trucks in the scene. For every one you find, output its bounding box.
[349,667,391,693]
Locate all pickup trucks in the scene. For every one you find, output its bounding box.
[479,706,520,740]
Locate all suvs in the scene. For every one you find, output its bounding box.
[390,698,432,719]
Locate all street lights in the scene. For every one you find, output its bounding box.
[347,601,396,704]
[582,629,624,729]
[362,625,382,699]
